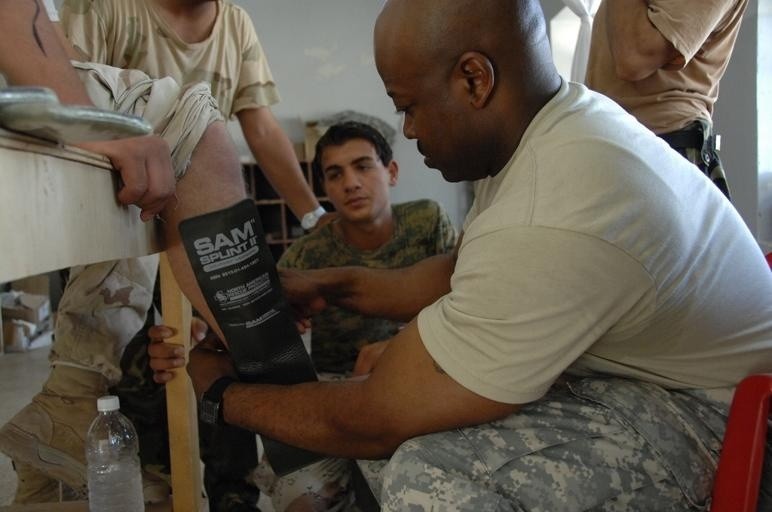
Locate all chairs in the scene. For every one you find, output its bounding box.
[708,376,772,512]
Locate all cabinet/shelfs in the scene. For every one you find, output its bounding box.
[242,159,329,251]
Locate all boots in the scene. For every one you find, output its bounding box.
[0,366,172,504]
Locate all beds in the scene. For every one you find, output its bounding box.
[0,126,210,512]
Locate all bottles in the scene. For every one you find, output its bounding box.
[85,394,145,512]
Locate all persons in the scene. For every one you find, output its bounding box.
[185,1,772,512]
[0,0,349,512]
[277,120,459,383]
[583,0,748,202]
